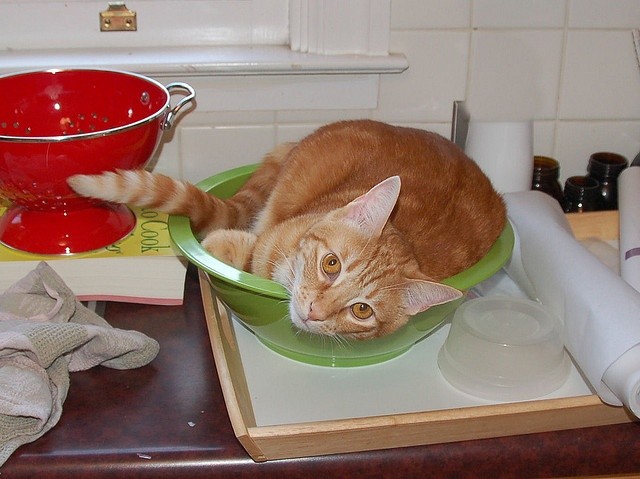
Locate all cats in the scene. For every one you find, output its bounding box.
[65,118,508,352]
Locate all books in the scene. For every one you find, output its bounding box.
[0,201,189,310]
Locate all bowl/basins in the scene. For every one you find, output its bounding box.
[436,296,571,402]
[167,162,515,368]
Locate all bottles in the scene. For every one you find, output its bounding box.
[586,151,629,213]
[530,156,563,203]
[564,176,601,212]
